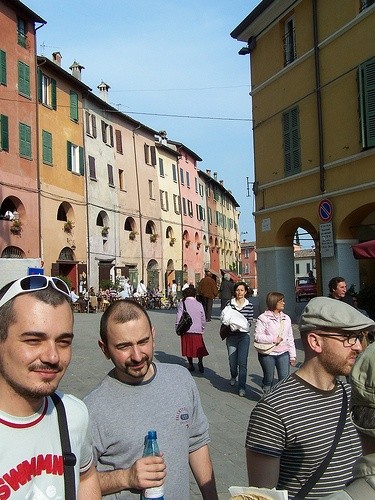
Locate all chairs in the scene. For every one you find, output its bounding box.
[70,288,173,314]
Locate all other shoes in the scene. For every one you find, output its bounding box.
[187,365,195,372]
[198,362,204,373]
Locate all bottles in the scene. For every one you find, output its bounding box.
[141,430,165,500]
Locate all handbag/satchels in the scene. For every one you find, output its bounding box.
[176,311,192,336]
[254,341,276,355]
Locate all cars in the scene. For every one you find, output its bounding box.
[296,277,318,303]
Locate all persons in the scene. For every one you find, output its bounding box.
[174,287,211,373]
[316,341,375,500]
[82,299,219,500]
[0,275,105,500]
[221,282,254,397]
[253,291,297,393]
[70,277,188,313]
[352,296,370,318]
[307,270,314,283]
[218,273,236,311]
[197,271,216,323]
[327,276,354,306]
[245,296,363,500]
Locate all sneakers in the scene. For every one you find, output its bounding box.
[239,388,245,397]
[230,377,235,386]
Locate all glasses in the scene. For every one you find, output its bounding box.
[315,332,364,345]
[0,275,73,309]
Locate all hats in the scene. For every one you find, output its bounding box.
[299,297,375,336]
[349,340,375,439]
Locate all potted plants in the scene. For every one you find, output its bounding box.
[9,219,23,235]
[63,220,191,248]
